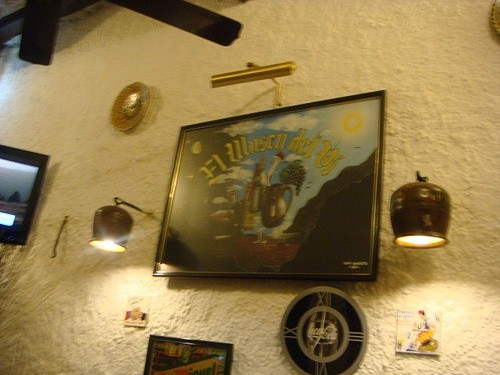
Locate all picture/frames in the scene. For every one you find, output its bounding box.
[0,145,48,246]
[151,89,385,279]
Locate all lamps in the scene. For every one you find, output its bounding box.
[88,197,150,252]
[390,171,450,250]
